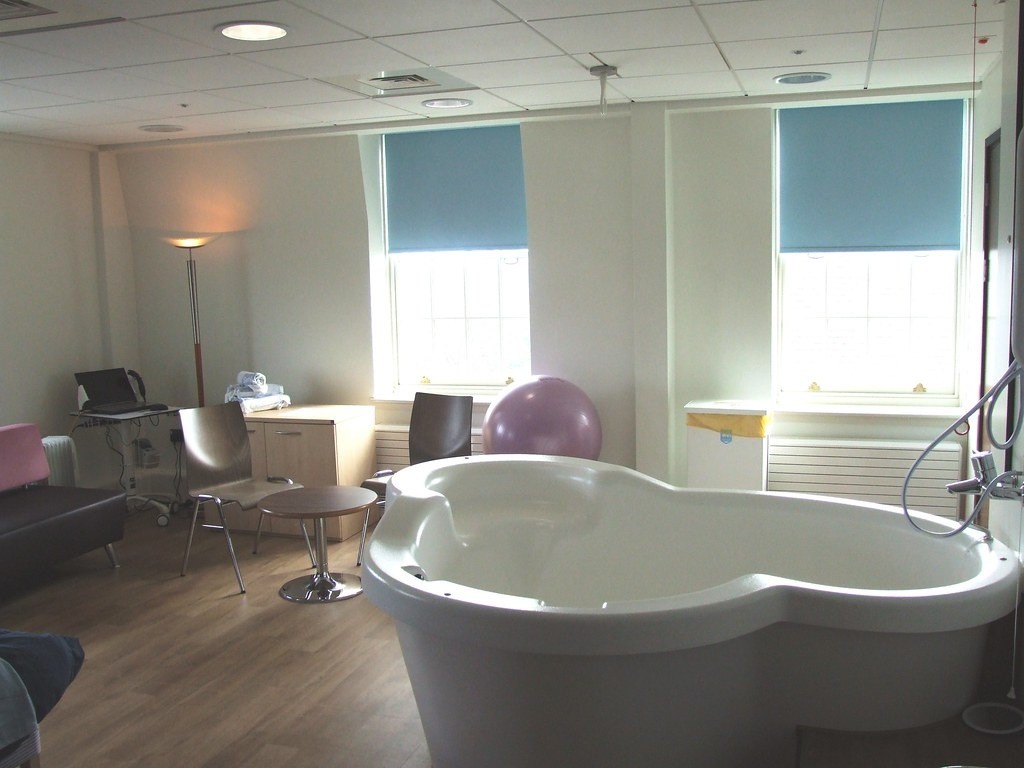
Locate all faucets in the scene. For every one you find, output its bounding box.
[946,450,1022,502]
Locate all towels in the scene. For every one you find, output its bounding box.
[223,369,293,415]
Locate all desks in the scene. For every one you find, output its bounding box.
[70,403,188,528]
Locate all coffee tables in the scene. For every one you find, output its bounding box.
[256,485,379,605]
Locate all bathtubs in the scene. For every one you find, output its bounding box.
[355,449,1022,768]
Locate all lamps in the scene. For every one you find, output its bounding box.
[160,234,215,409]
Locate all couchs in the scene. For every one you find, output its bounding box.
[1,422,128,591]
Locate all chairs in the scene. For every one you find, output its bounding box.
[357,390,474,566]
[177,400,317,594]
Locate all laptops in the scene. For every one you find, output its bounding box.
[74,367,156,416]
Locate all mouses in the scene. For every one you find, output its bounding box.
[150,404,168,411]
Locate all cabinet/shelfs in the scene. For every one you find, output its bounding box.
[684,399,769,492]
[201,403,377,544]
